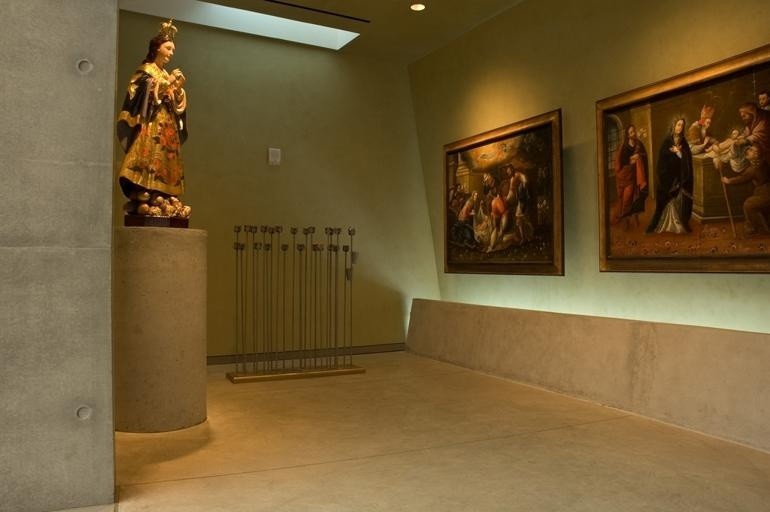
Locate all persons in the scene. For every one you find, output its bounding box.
[614,93,770,233]
[118,32,188,199]
[450,164,536,254]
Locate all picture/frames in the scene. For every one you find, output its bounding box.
[594,43,770,275]
[442,107,565,276]
